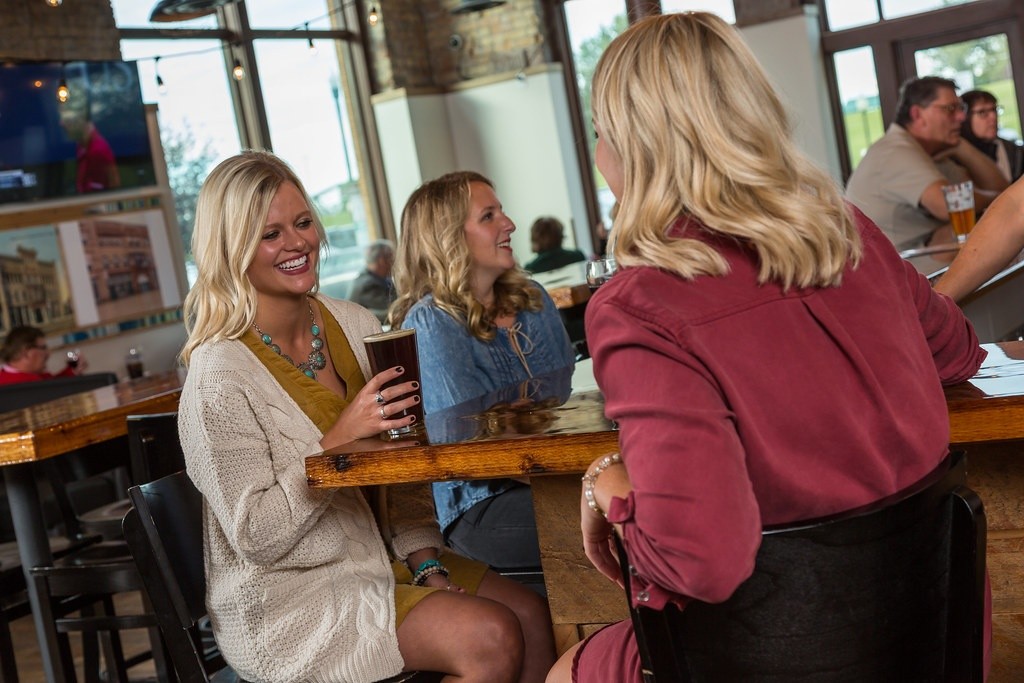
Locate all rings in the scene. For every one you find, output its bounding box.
[380,404,388,419]
[376,392,387,404]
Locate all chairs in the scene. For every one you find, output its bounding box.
[29,409,242,683]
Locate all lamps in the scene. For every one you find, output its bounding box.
[149,0,226,21]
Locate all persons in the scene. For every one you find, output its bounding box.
[0,326,88,386]
[347,241,398,324]
[523,217,581,276]
[388,171,578,567]
[61,105,123,192]
[545,11,996,683]
[844,75,1024,301]
[175,149,555,683]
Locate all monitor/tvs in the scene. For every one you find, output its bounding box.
[0,57,159,205]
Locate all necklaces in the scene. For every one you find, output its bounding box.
[252,297,326,381]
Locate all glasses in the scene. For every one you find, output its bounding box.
[921,102,968,116]
[970,106,1003,118]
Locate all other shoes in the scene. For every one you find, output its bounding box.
[45,510,85,537]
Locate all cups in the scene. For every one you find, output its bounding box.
[941,180,976,245]
[586,259,620,294]
[64,349,81,369]
[125,351,143,379]
[363,328,426,437]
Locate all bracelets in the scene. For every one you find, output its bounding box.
[582,453,623,518]
[410,560,451,587]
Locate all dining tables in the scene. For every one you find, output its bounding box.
[302,338,1024,682]
[0,363,197,683]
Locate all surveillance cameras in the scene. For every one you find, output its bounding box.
[448,34,462,50]
